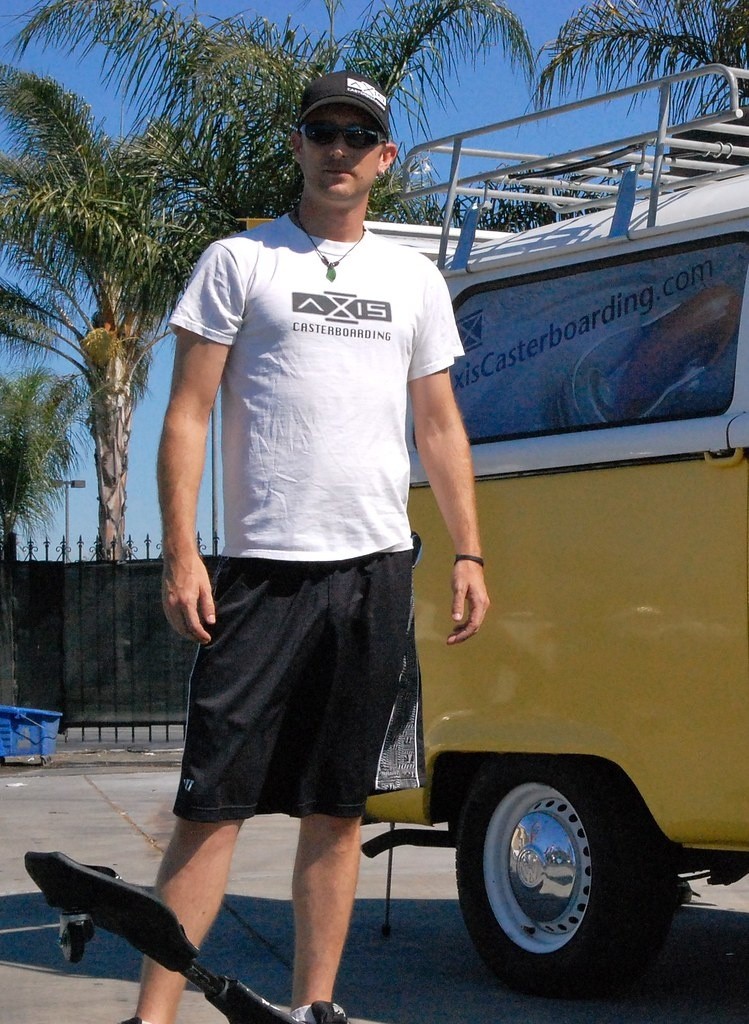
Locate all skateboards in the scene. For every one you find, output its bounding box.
[22,846,355,1023]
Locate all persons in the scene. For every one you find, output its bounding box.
[129,68,492,1024]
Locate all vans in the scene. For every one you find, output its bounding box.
[232,64,748,996]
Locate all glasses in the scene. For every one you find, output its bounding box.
[299,121,388,148]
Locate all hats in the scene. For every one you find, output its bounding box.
[297,69,389,135]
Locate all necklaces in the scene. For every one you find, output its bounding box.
[295,206,365,280]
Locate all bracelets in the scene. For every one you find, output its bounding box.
[452,555,489,567]
